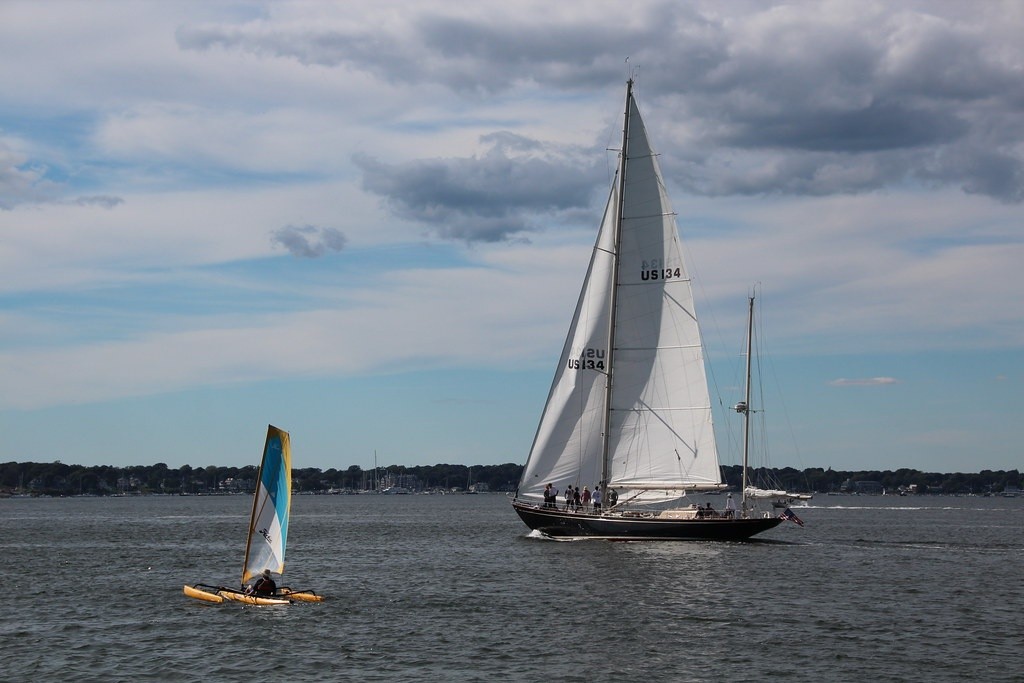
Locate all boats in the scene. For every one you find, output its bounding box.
[770,501,791,509]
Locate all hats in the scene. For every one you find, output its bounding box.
[727,494,731,496]
[262,569,272,577]
[584,486,588,490]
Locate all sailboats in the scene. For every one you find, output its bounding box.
[502,78,786,540]
[183,423,325,606]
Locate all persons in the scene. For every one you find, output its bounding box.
[610,488,618,508]
[245,569,277,597]
[574,487,583,513]
[564,484,574,512]
[591,486,602,515]
[581,486,591,512]
[544,483,559,508]
[695,503,719,520]
[721,493,736,516]
